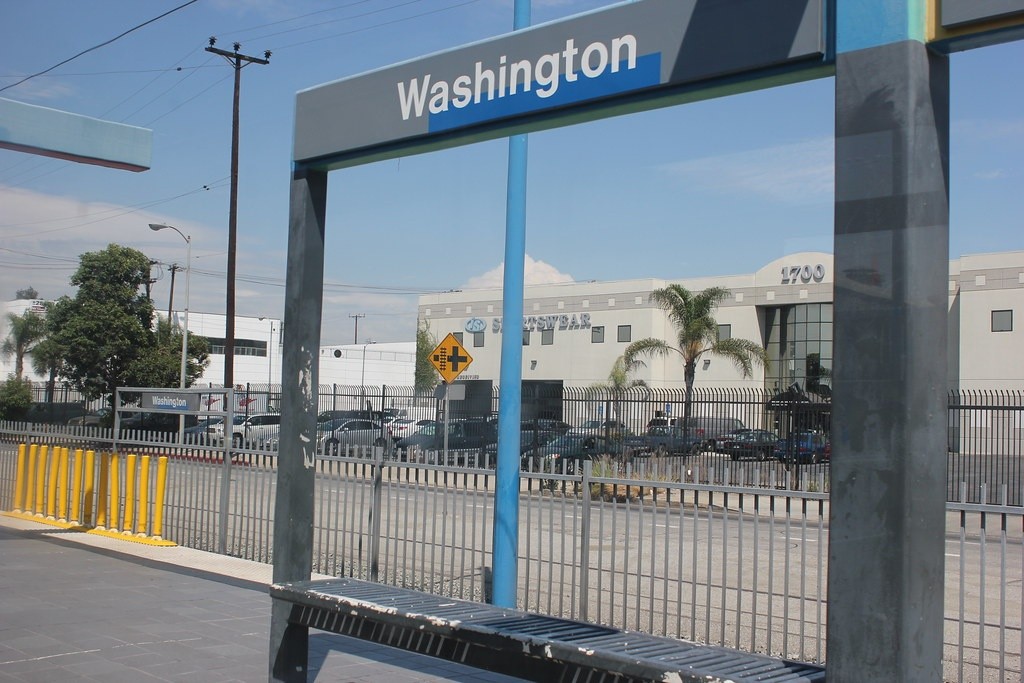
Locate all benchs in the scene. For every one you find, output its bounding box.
[268,574,827,683]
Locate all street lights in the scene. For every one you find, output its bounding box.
[149,222,191,446]
[361,340,376,413]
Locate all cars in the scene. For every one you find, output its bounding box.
[67,407,830,476]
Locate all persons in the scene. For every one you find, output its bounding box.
[366,400,372,412]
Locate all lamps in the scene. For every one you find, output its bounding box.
[704,360,710,364]
[531,360,537,364]
